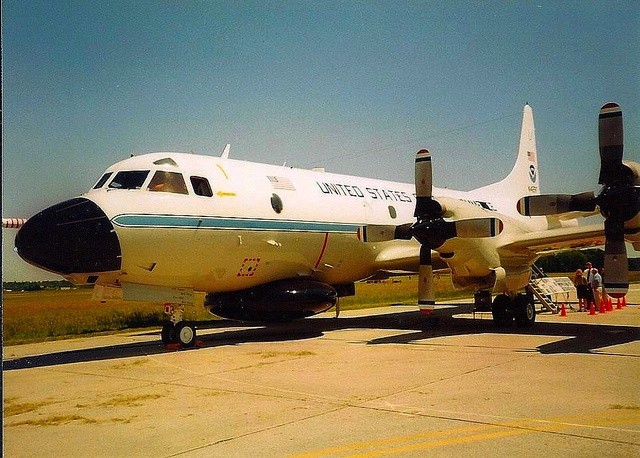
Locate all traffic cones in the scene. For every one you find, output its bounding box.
[622,296,626,306]
[609,298,612,309]
[561,303,566,316]
[599,299,605,313]
[606,299,610,311]
[589,302,595,315]
[617,298,621,309]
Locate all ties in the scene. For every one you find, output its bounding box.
[587,270,590,282]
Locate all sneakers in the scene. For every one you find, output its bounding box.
[584,308,588,312]
[577,308,582,312]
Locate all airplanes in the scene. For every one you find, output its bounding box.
[12,101,583,350]
[355,148,600,327]
[516,102,640,299]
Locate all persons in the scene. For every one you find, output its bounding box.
[591,269,603,310]
[600,268,607,300]
[538,267,551,302]
[583,262,595,310]
[574,269,588,312]
